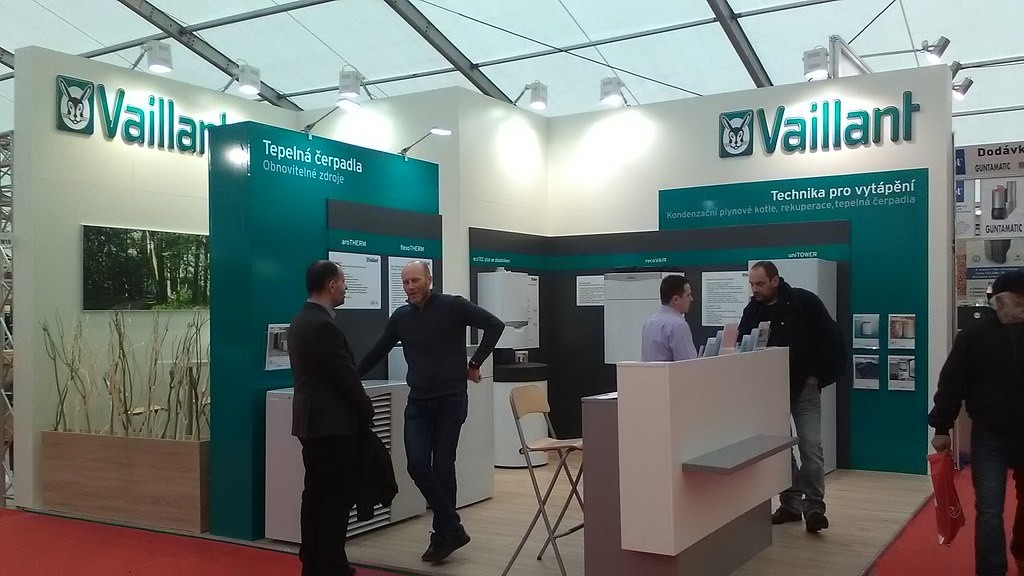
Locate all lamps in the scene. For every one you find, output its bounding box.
[857,35,952,62]
[513,78,551,113]
[399,125,455,162]
[129,37,176,75]
[952,74,978,99]
[338,63,378,101]
[219,57,268,97]
[951,59,965,82]
[801,43,834,83]
[598,74,636,110]
[301,97,365,142]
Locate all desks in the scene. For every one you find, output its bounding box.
[156,359,209,438]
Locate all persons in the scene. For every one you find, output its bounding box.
[735,261,849,532]
[641,274,697,362]
[928,270,1024,576]
[354,261,506,565]
[287,259,375,576]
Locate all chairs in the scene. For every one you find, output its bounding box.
[499,381,585,576]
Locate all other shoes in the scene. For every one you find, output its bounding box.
[422,525,471,564]
[806,510,829,533]
[772,508,803,524]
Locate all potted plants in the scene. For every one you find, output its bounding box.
[27,296,211,534]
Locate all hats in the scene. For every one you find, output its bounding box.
[991,270,1024,296]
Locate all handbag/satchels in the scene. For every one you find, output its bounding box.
[927,450,967,544]
